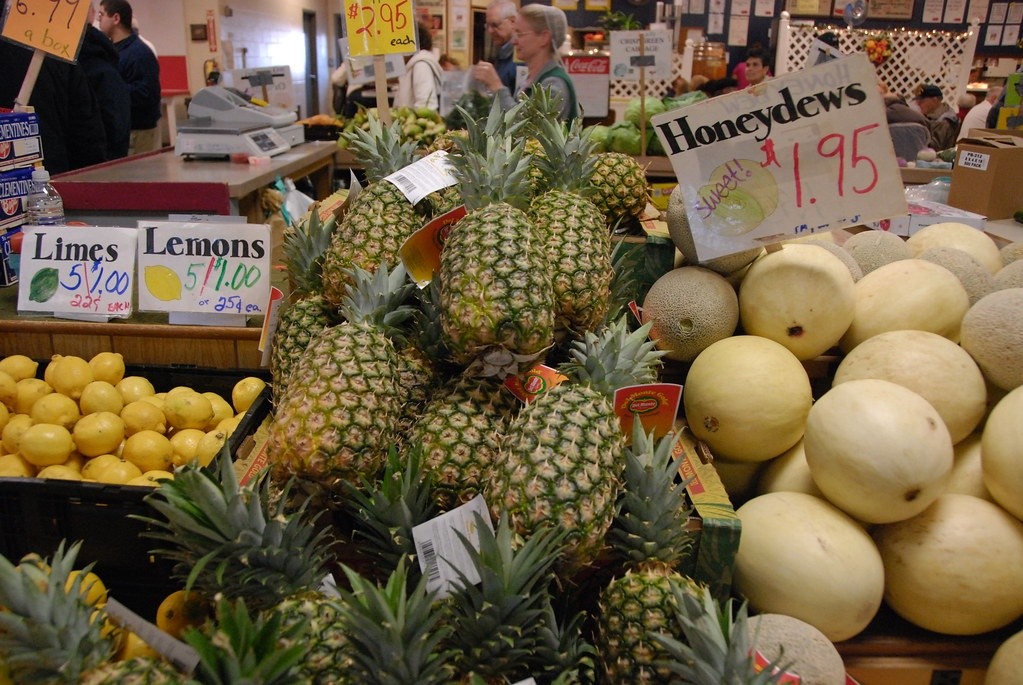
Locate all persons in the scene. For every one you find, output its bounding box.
[0,0,162,175]
[476,0,581,140]
[393,23,441,117]
[332,61,368,113]
[876,85,1003,153]
[732,44,773,92]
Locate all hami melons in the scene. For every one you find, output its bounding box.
[640,181,1023,685]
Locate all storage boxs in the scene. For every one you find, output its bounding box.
[946,136,1023,221]
[0,357,274,626]
[864,200,988,237]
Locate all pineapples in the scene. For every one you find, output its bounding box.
[0,537,212,685]
[514,81,564,191]
[102,129,797,685]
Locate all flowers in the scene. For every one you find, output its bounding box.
[865,35,892,64]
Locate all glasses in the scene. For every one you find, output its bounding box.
[483,18,510,30]
[515,30,540,39]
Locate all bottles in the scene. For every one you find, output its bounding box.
[27,171,65,229]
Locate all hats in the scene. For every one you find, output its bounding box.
[912,83,943,101]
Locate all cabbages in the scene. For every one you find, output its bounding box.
[581,91,713,158]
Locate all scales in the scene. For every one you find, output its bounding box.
[175,120,290,163]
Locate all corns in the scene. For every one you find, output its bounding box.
[337,107,439,146]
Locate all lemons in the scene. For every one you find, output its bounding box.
[0,352,266,660]
